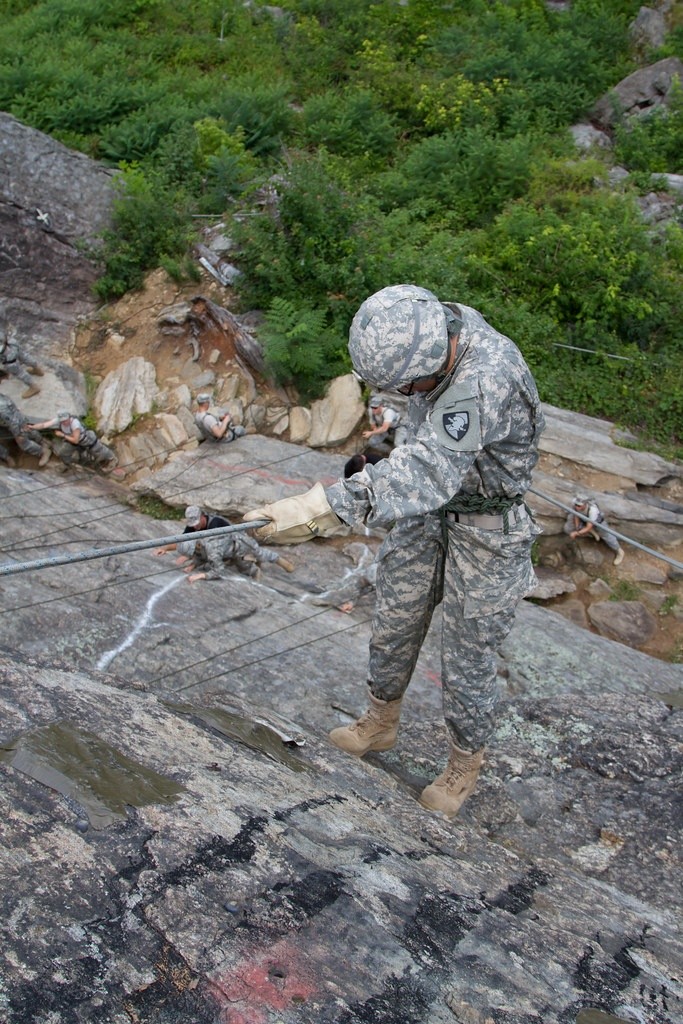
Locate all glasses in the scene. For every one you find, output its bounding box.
[396,380,415,396]
[371,407,378,409]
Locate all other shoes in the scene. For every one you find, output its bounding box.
[251,568,261,583]
[246,427,256,434]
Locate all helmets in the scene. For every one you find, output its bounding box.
[347,283,449,392]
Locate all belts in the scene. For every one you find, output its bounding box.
[438,503,528,531]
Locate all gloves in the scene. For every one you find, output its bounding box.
[242,481,343,544]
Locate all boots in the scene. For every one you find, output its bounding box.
[590,528,600,542]
[277,557,294,572]
[27,366,44,376]
[418,740,486,818]
[22,381,40,398]
[38,447,51,466]
[614,547,624,565]
[40,438,52,449]
[330,690,403,758]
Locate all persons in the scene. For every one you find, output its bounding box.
[244,286,544,816]
[571,493,627,566]
[0,331,44,399]
[363,396,408,456]
[158,506,233,565]
[22,409,120,470]
[344,452,383,479]
[195,393,245,443]
[175,528,294,581]
[0,396,53,467]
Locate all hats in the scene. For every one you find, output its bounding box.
[57,409,69,422]
[369,397,383,408]
[185,505,201,527]
[176,538,196,559]
[196,394,210,402]
[574,494,589,506]
